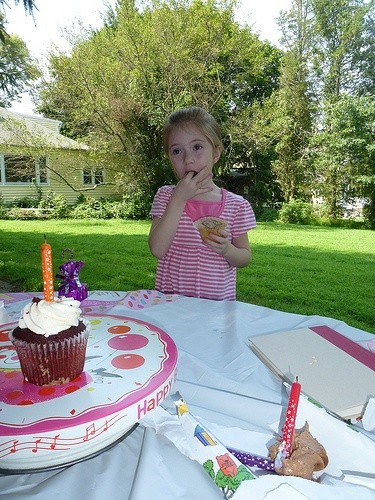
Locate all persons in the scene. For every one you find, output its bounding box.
[149,106,260,300]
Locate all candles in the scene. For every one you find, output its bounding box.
[40,233,56,302]
[226,446,276,473]
[279,373,303,455]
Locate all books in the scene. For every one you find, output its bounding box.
[249,325,375,419]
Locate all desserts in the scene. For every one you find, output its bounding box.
[268,431,328,480]
[8,296,91,387]
[192,216,229,244]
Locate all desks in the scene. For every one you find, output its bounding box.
[1,290,374,500]
